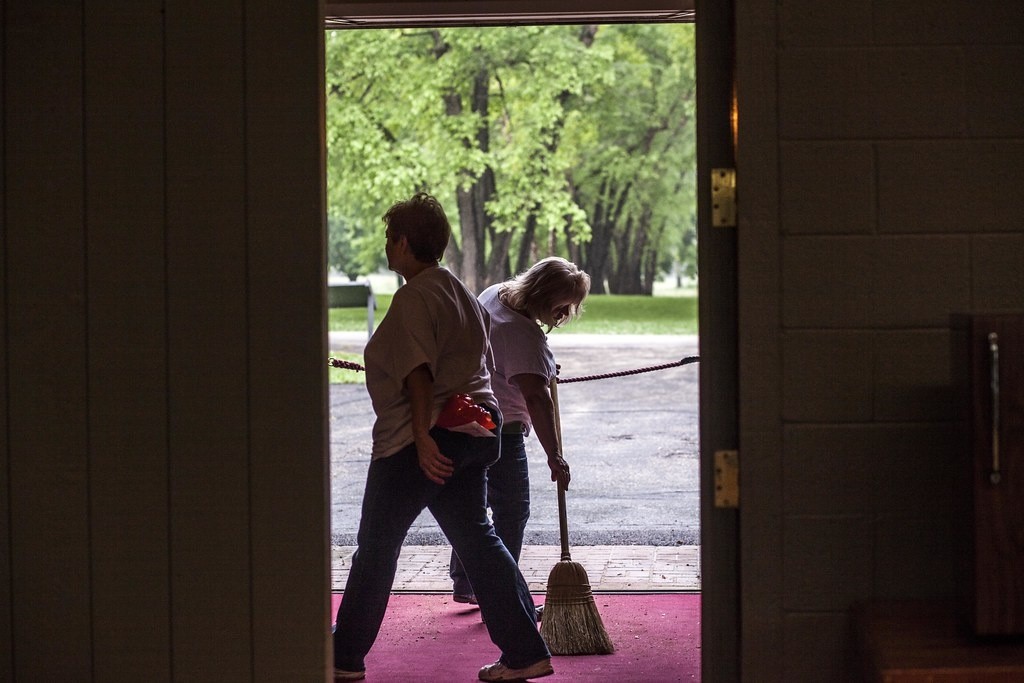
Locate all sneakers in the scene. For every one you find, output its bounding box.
[334,659,366,679]
[535,604,546,624]
[454,586,476,604]
[478,654,554,681]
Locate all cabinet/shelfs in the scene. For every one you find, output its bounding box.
[849,599,1024,683]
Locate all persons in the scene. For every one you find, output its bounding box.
[332,194,554,681]
[450,254,591,627]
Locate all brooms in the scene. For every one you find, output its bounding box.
[539,378,616,657]
